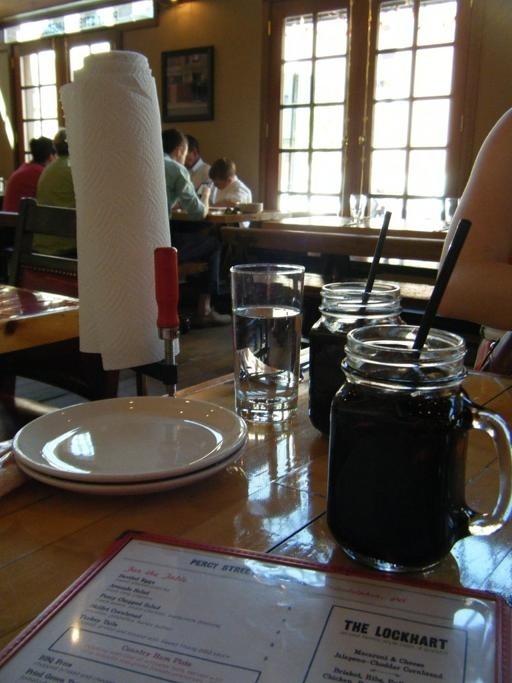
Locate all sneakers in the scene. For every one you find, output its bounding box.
[192,309,232,328]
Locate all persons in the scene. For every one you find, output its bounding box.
[434,105,512,334]
[4,129,78,259]
[162,129,252,327]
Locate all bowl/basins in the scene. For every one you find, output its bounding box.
[235,202,263,214]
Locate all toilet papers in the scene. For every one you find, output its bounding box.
[55,49,182,374]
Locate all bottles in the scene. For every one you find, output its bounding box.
[323,321,512,576]
[307,280,409,434]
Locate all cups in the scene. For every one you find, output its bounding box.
[444,197,463,229]
[349,193,368,227]
[230,261,306,419]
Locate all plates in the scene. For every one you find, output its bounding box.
[11,395,250,483]
[12,435,249,497]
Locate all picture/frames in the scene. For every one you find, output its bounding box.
[161,42,216,122]
